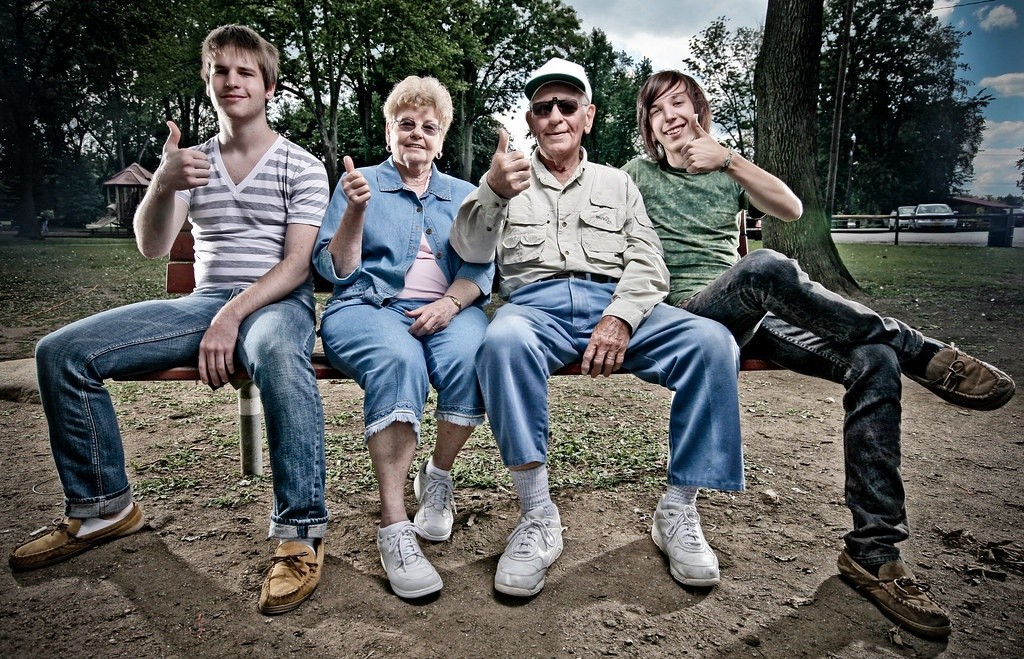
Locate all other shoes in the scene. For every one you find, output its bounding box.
[258,538,325,614]
[899,336,1016,411]
[836,548,953,634]
[8,502,144,572]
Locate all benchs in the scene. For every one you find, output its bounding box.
[110,215,787,478]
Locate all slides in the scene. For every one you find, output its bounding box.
[85,214,117,229]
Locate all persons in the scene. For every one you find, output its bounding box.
[622,73,1017,634]
[312,76,498,599]
[10,25,329,615]
[449,57,744,596]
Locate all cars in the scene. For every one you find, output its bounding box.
[868,204,959,231]
[1001,209,1024,226]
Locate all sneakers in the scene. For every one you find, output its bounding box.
[414,459,457,541]
[651,494,720,587]
[494,504,567,595]
[377,520,443,598]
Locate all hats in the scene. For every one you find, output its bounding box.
[524,57,593,103]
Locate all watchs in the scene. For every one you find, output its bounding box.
[443,295,461,314]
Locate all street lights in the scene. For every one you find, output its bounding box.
[845,134,860,206]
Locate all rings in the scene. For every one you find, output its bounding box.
[432,326,437,332]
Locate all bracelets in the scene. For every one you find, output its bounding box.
[719,149,732,173]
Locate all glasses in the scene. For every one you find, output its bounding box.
[390,117,443,136]
[530,96,590,117]
